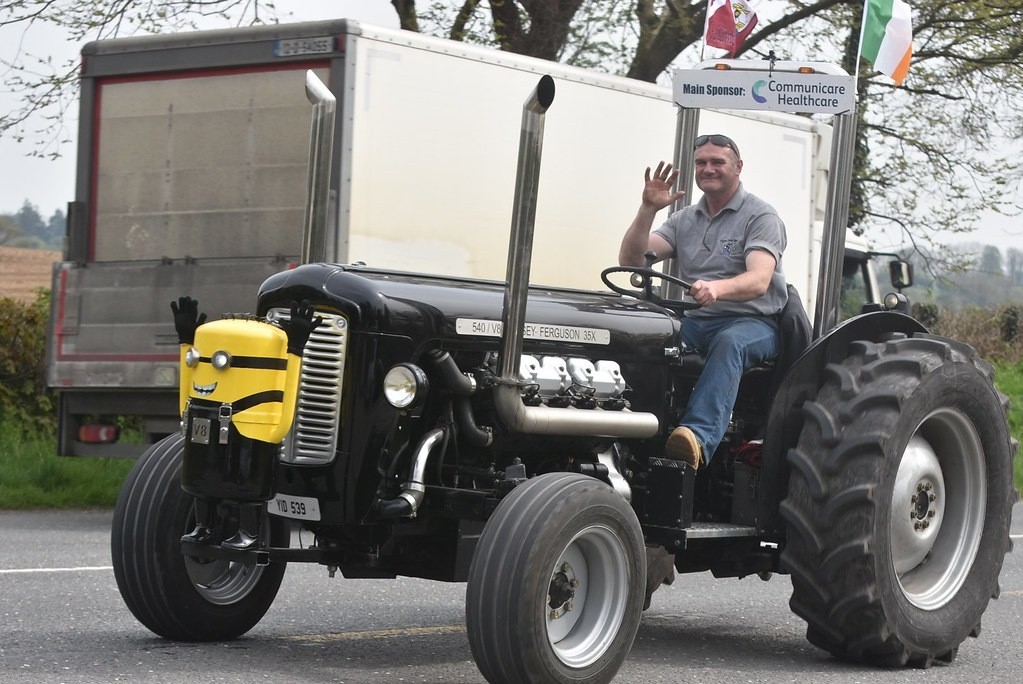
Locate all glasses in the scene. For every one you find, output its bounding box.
[693,134,740,160]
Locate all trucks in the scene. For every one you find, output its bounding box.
[38,15,915,479]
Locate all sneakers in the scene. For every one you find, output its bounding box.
[666,426,704,476]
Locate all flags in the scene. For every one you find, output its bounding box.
[861,0,912,84]
[705,0,758,58]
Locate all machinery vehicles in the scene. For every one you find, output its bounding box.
[110,72,1022,684]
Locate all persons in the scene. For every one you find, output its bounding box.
[618,134,789,469]
